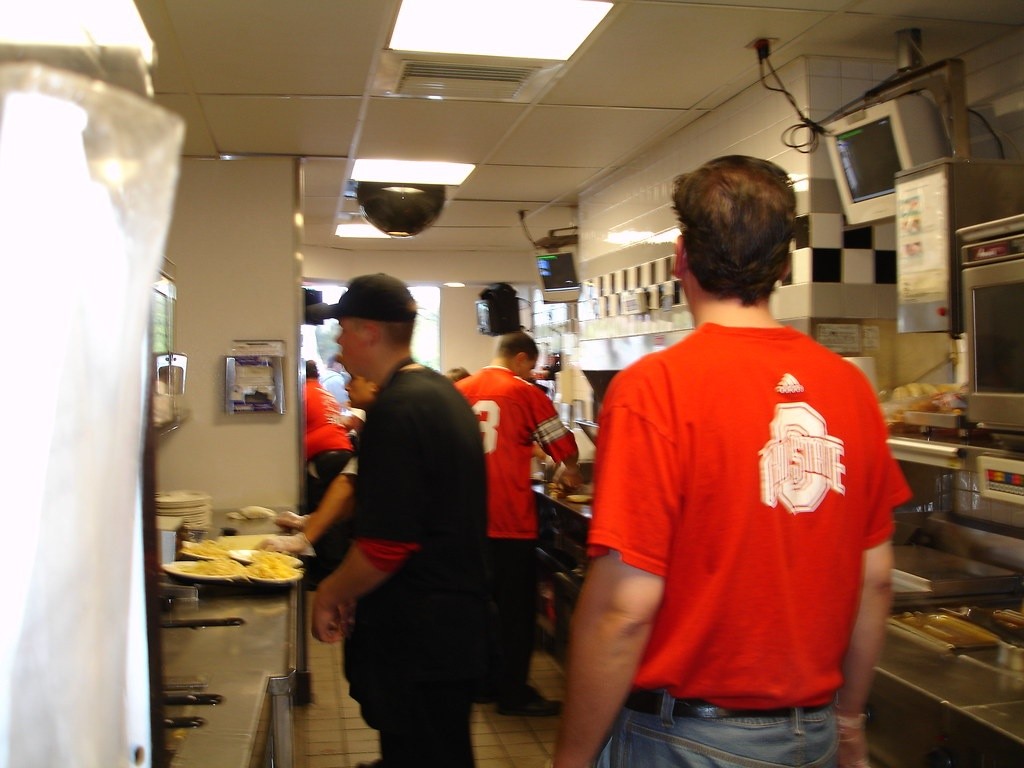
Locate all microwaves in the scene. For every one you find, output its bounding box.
[148,274,188,433]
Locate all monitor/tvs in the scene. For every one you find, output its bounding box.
[533,244,581,304]
[822,93,947,227]
[961,259,1024,434]
[475,282,521,337]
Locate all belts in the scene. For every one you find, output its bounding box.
[626,690,830,718]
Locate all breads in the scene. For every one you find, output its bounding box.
[890,383,960,402]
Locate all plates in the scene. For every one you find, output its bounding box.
[229,549,303,566]
[161,561,241,580]
[155,491,211,530]
[179,549,224,560]
[245,566,303,582]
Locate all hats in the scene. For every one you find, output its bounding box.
[307,272,416,322]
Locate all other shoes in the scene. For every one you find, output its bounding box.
[473,693,497,703]
[508,696,562,716]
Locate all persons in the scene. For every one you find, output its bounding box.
[552,154,914,768]
[445,332,579,718]
[255,273,487,768]
